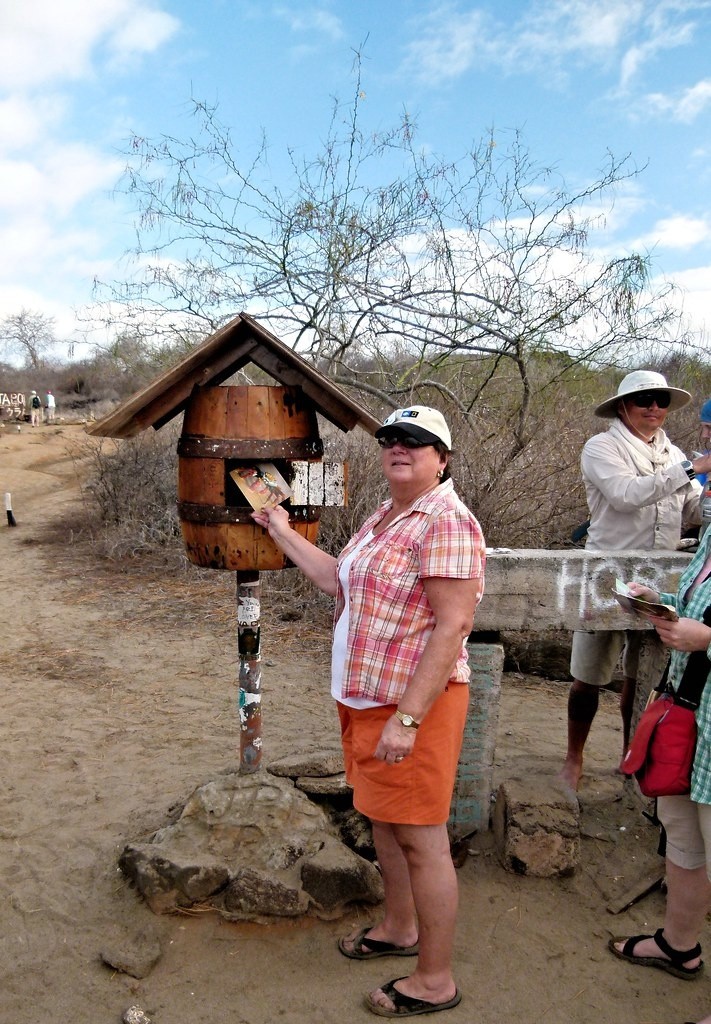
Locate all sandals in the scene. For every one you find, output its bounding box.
[608,928,705,981]
[683,1022,696,1023]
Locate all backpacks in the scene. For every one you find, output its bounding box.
[32,396,40,409]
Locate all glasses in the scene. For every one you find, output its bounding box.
[378,435,434,449]
[625,393,672,409]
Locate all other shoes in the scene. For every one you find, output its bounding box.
[32,424,39,427]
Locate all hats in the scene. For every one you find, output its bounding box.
[47,390,51,394]
[699,399,711,422]
[374,405,452,450]
[594,370,692,419]
[32,390,36,394]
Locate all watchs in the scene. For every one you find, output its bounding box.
[395,710,420,729]
[680,460,695,480]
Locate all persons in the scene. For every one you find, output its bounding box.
[27,390,42,427]
[251,404,486,1017]
[607,521,711,1024]
[556,371,711,791]
[45,389,56,426]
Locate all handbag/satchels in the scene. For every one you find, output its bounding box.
[619,685,697,798]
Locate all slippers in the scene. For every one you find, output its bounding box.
[338,927,419,959]
[364,976,462,1018]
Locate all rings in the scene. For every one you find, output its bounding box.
[396,757,403,760]
